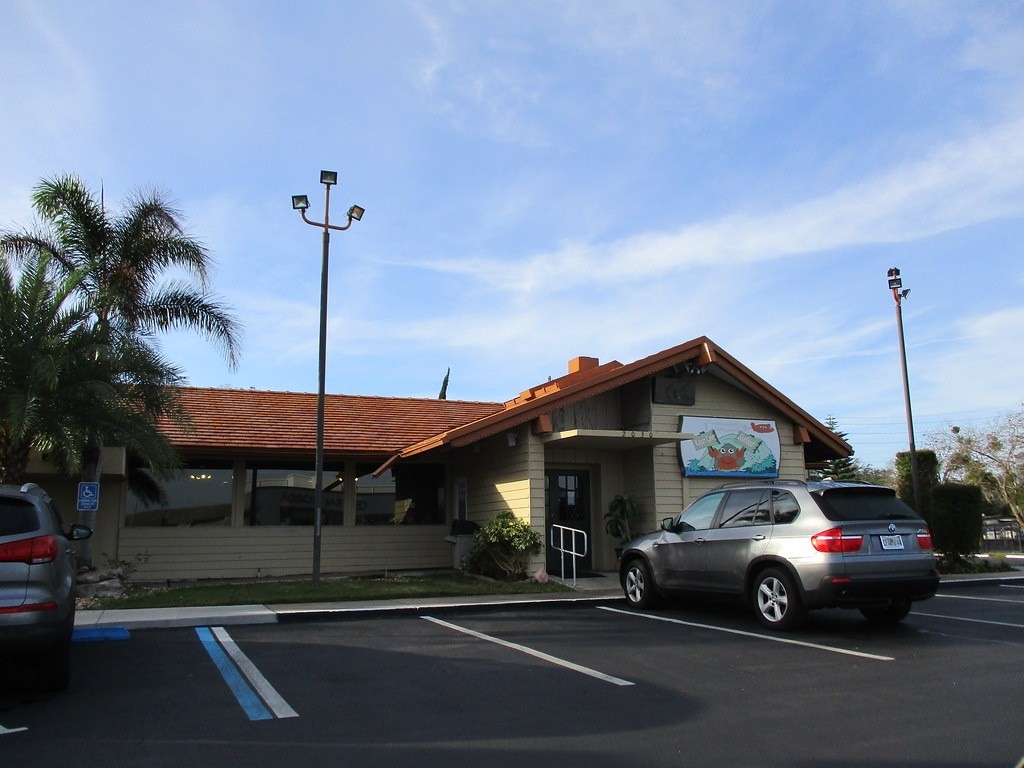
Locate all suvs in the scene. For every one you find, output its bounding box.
[618,478,941,633]
[0,483,93,696]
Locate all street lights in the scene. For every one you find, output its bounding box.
[292,170,364,587]
[888,268,923,518]
